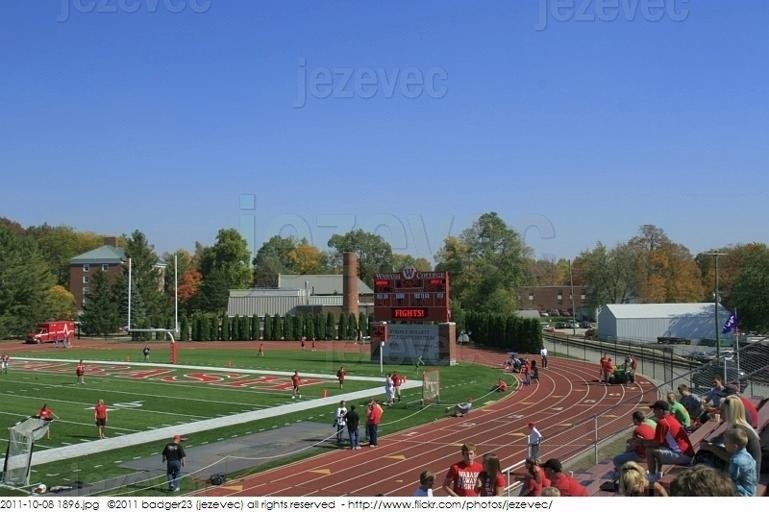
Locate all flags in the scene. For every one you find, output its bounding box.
[722,312,739,334]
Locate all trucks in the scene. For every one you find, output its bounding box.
[24,319,75,343]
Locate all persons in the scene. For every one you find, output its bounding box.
[301,337,305,349]
[143,345,150,360]
[162,435,186,491]
[76,359,87,384]
[415,421,592,496]
[335,399,383,450]
[311,337,316,352]
[291,370,303,399]
[337,367,346,388]
[256,341,264,358]
[414,354,424,375]
[37,403,59,439]
[600,352,636,385]
[494,346,548,392]
[386,370,406,404]
[0,352,9,375]
[95,399,108,439]
[446,397,472,414]
[613,374,761,497]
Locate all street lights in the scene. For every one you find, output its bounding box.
[380,341,386,374]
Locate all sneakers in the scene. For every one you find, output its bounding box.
[645,470,663,483]
[173,487,181,492]
[335,438,378,451]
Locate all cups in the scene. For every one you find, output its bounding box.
[714,414,720,422]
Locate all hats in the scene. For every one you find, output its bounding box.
[649,400,670,411]
[722,384,739,393]
[539,459,563,469]
[173,435,180,441]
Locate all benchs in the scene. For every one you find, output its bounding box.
[566,393,769,496]
